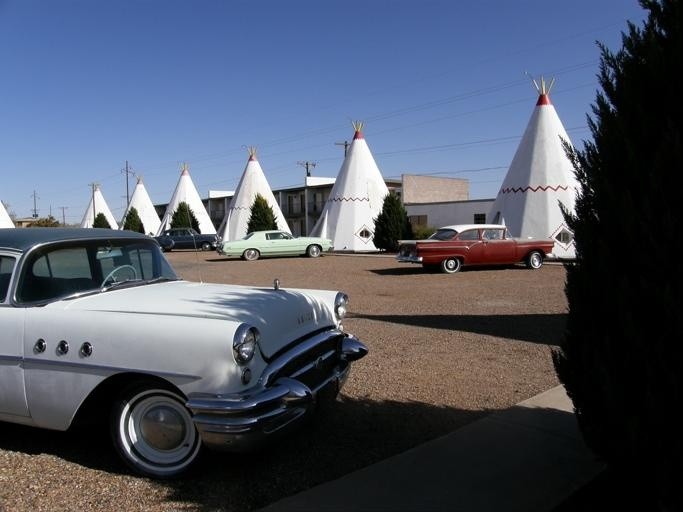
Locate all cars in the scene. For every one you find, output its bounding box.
[217,230,334,262]
[395,223,554,274]
[0,227,369,481]
[152,227,219,252]
